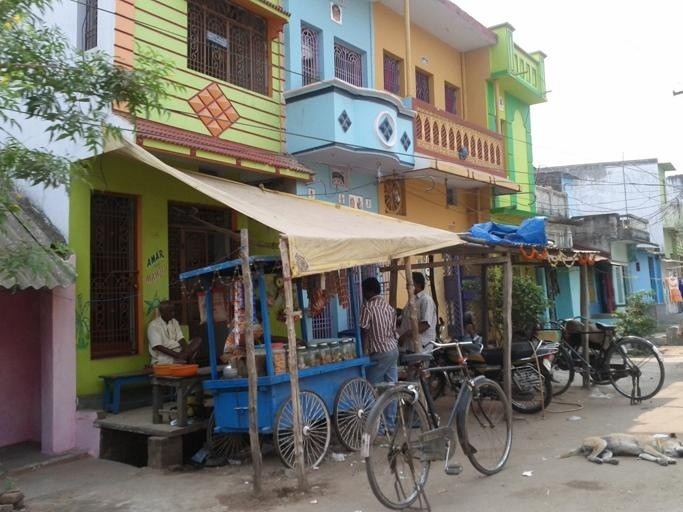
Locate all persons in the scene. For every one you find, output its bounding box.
[361,275,398,436]
[147,299,203,366]
[398,270,437,426]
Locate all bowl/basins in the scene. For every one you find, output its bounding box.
[153,364,199,378]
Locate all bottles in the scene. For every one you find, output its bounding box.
[252,337,356,377]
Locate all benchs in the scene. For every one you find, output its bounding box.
[98,364,227,428]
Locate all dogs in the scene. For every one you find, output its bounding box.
[556,432,683,466]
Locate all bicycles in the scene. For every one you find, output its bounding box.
[359,334,514,512]
[532,315,666,407]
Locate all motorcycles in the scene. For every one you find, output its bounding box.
[425,317,565,414]
